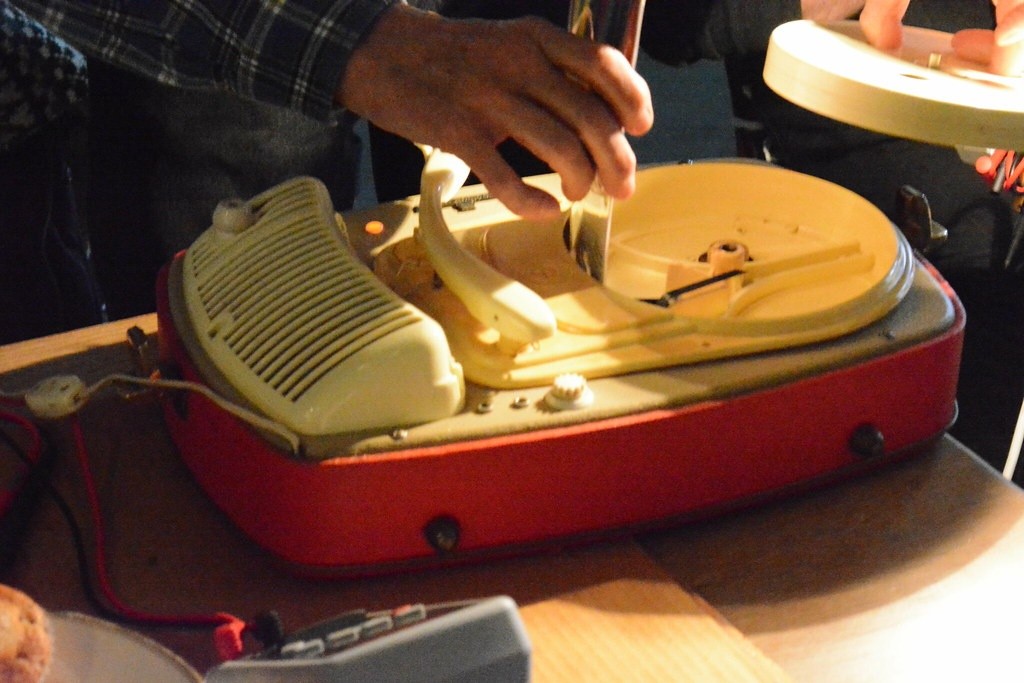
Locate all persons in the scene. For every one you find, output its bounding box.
[1,1,1023,494]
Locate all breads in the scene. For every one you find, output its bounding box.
[0,585,56,683]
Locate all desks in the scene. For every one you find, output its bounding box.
[0,314,1024,683]
[763,18,1024,152]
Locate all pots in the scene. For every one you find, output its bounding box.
[156,154,969,578]
